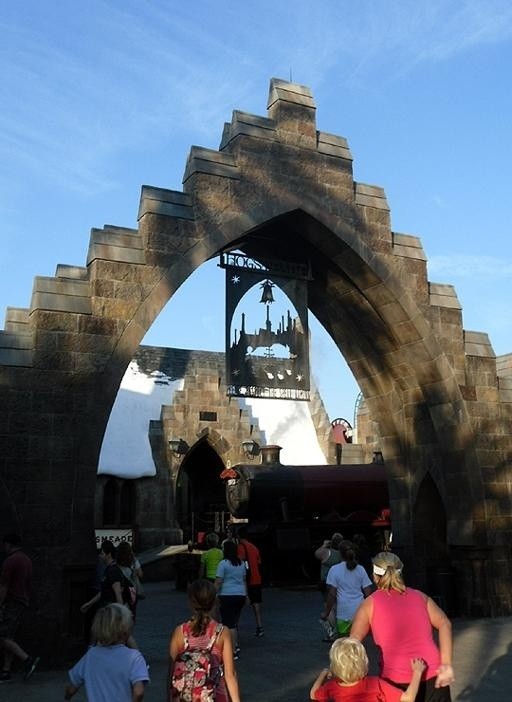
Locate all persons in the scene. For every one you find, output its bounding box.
[314,532,344,643]
[63,524,267,702]
[349,550,455,702]
[0,533,41,684]
[309,635,428,702]
[319,540,373,638]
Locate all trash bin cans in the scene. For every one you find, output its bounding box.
[174,553,201,592]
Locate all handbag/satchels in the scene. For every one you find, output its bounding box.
[243,558,251,577]
[123,576,137,606]
[131,569,146,600]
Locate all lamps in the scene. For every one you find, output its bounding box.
[168,437,183,459]
[242,439,255,460]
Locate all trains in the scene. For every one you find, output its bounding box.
[225,439,392,588]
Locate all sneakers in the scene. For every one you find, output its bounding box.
[21,656,41,682]
[0,674,11,683]
[256,629,264,637]
[233,646,241,660]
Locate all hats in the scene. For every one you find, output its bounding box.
[115,541,134,566]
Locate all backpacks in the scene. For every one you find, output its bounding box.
[171,621,224,702]
